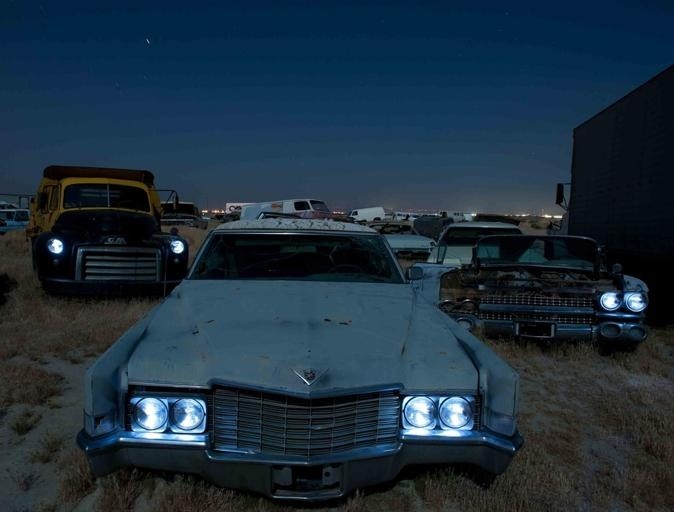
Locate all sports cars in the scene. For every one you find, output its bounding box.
[74,215,524,502]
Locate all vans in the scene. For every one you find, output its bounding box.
[0,209,30,233]
[239,198,332,224]
[0,201,19,209]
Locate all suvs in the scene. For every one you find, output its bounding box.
[28,164,191,295]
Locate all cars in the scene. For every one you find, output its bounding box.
[160,200,208,230]
